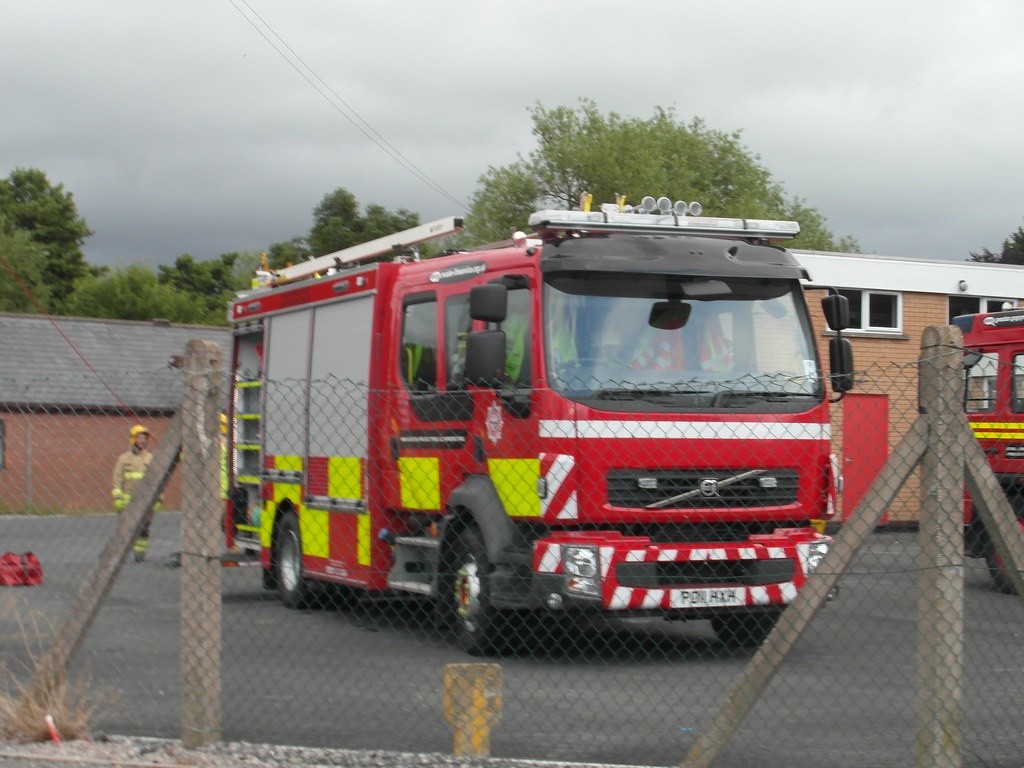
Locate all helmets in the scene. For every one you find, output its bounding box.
[130,424,149,445]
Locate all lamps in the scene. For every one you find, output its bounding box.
[959,280,967,291]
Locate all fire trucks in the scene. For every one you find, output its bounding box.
[948,308,1024,597]
[218,192,855,659]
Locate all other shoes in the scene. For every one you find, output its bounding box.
[135,557,144,564]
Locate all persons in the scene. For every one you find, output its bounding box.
[112,425,166,565]
[631,291,740,385]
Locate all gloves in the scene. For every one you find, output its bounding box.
[113,498,124,514]
[152,501,162,512]
